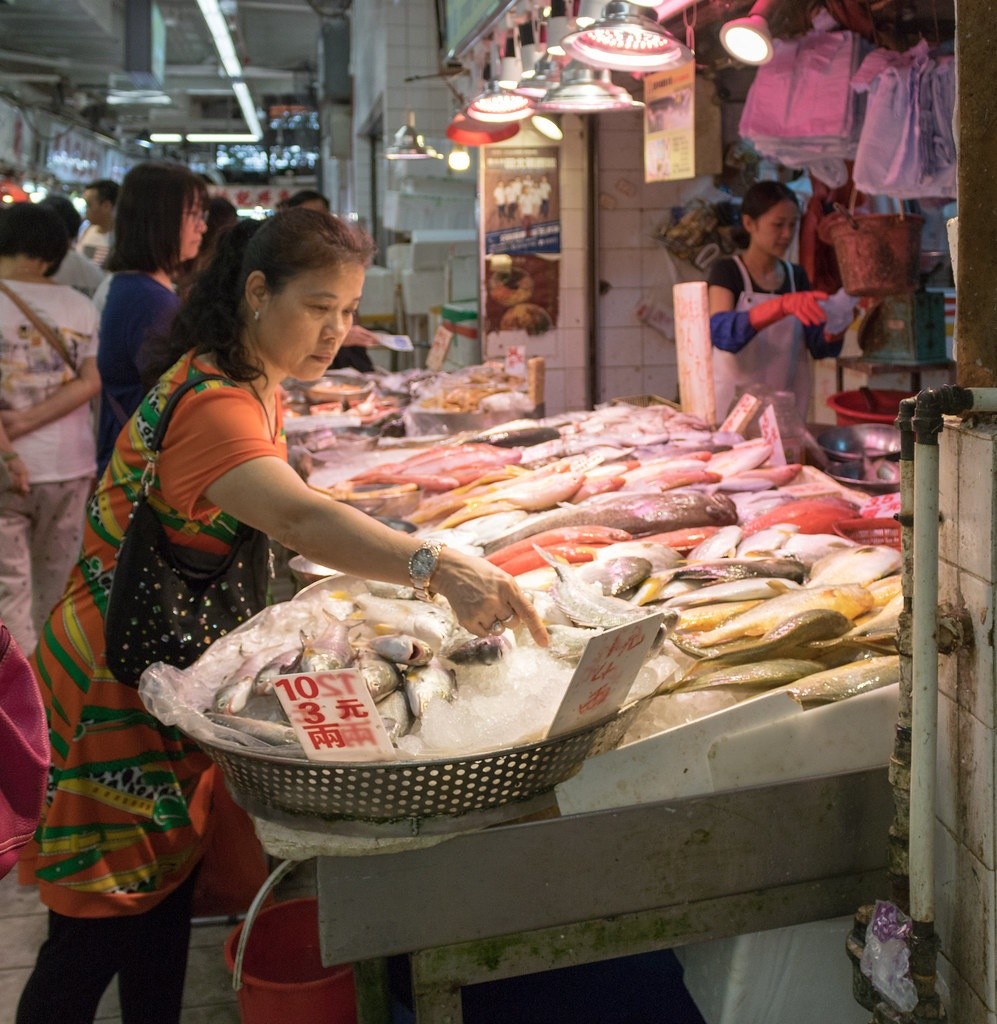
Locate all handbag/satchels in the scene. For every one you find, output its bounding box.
[0,622,52,878]
[103,373,273,687]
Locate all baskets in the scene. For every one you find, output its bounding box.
[178,686,667,822]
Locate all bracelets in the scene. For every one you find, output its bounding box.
[2,451,16,461]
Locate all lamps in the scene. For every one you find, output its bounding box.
[383,0,776,171]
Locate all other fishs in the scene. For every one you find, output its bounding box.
[208,400,906,760]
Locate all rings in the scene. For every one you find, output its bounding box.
[486,621,502,631]
[501,614,514,623]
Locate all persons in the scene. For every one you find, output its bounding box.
[0,194,101,657]
[273,188,337,212]
[708,178,861,427]
[96,155,384,482]
[647,96,678,132]
[74,179,123,274]
[14,207,550,1024]
[176,196,238,293]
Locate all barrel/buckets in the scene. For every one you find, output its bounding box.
[824,188,927,297]
[826,389,918,427]
[226,860,357,1024]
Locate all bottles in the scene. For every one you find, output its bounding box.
[726,380,809,467]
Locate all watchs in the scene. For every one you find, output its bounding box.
[408,538,448,604]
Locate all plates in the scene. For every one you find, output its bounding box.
[489,266,553,331]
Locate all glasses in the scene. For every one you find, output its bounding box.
[183,209,209,228]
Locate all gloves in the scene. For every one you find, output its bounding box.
[749,289,829,334]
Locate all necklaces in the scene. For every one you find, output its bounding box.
[248,377,274,444]
[158,272,177,288]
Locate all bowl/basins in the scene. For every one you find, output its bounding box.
[822,460,901,495]
[288,483,425,586]
[378,367,531,436]
[918,250,948,274]
[292,374,376,405]
[816,423,902,463]
[286,425,385,465]
